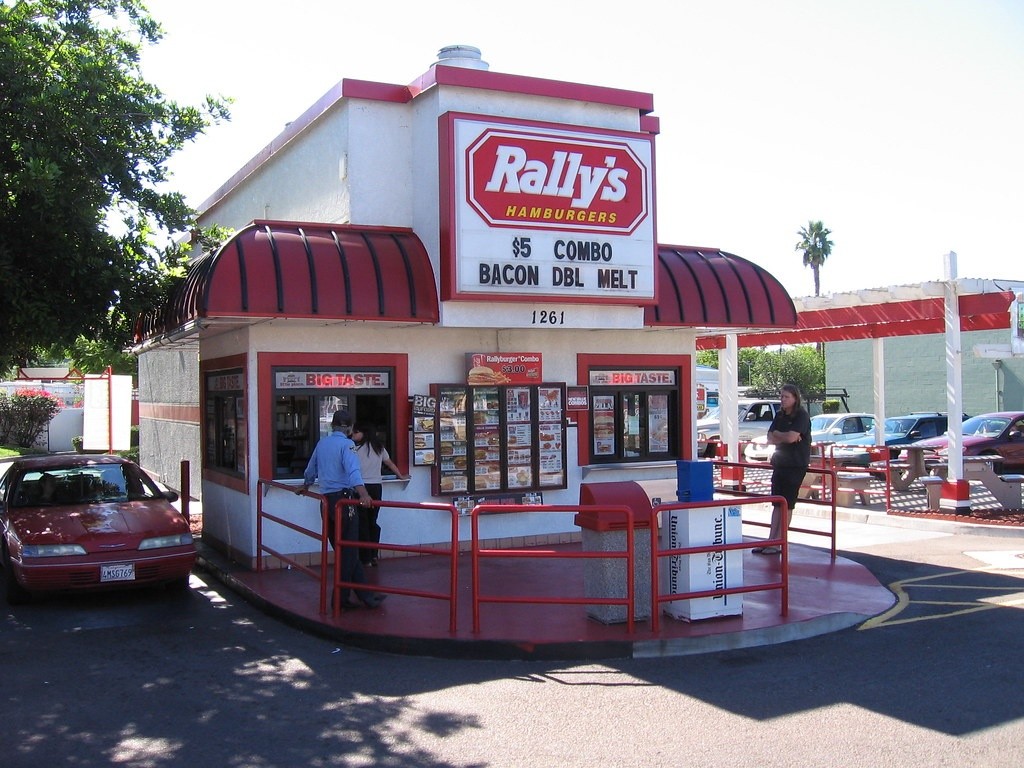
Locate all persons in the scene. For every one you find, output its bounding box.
[1010,424,1024,437]
[752,383,813,554]
[350,418,411,565]
[101,470,125,498]
[27,475,56,506]
[982,424,992,433]
[295,410,388,612]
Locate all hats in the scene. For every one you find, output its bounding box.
[333,410,351,427]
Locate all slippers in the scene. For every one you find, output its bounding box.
[751,546,766,554]
[762,547,782,554]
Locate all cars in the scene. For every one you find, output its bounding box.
[898,411,1024,481]
[0,454,199,601]
[827,411,972,473]
[743,414,898,465]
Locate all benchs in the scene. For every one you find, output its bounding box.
[834,458,1024,511]
[713,461,844,499]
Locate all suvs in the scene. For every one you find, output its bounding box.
[696,398,783,457]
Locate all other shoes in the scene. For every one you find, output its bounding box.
[364,593,387,608]
[331,599,360,609]
[360,556,377,568]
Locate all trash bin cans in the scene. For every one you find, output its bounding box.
[574,480,652,625]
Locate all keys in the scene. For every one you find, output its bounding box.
[348,509,355,517]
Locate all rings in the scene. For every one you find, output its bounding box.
[360,502,362,504]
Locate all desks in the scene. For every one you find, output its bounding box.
[887,443,944,491]
[797,455,842,500]
[941,455,1004,509]
